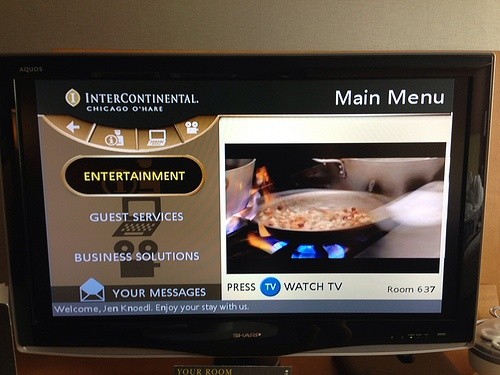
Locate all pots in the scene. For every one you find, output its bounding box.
[312,158,445,197]
[225,158,255,218]
[238,189,393,243]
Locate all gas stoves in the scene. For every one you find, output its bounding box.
[226,222,397,259]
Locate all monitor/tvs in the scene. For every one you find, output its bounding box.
[0,47,496,356]
